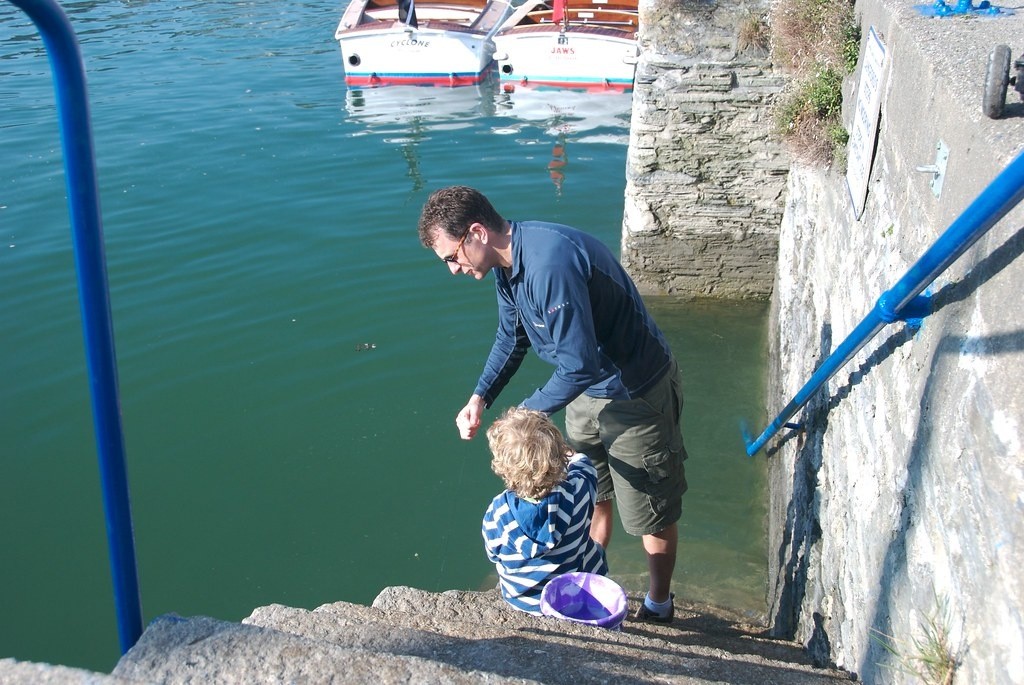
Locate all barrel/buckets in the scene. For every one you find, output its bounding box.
[539,572,629,630]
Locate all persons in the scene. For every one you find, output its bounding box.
[416,186,690,623]
[481,407,609,616]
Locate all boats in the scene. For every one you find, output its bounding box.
[345,79,486,148]
[492,79,633,197]
[490,0,640,98]
[333,0,520,88]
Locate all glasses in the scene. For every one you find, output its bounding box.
[442,222,484,263]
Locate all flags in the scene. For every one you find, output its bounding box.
[553,0,565,26]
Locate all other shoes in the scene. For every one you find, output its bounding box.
[634,591,675,626]
